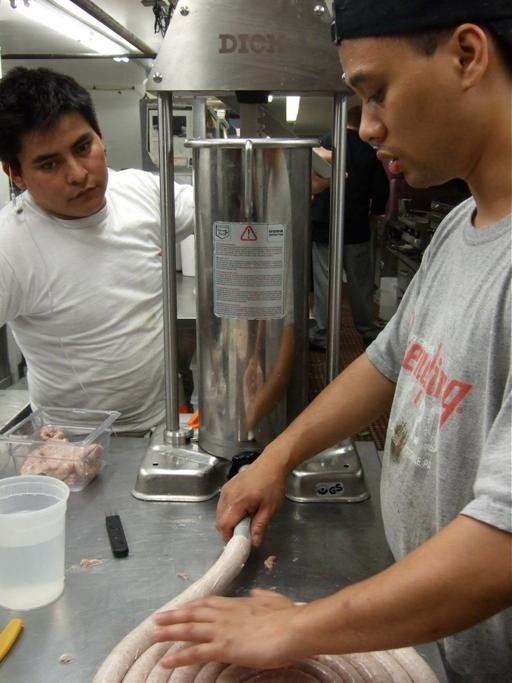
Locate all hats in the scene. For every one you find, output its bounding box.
[330,0,511,46]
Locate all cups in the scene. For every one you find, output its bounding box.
[0,471,71,611]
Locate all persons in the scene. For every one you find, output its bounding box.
[1,64,350,439]
[241,145,299,448]
[306,108,391,356]
[144,0,511,682]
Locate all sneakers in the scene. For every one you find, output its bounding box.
[309,328,328,352]
[364,332,377,343]
